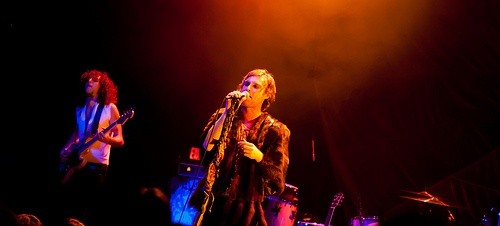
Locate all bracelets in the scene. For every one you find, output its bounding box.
[106,137,111,144]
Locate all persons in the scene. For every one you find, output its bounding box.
[59,70,125,210]
[202,68,292,226]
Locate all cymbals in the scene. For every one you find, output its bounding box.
[399,195,452,207]
[404,190,446,200]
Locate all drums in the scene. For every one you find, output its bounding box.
[280,183,298,201]
[263,195,298,226]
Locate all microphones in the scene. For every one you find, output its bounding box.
[226,90,250,100]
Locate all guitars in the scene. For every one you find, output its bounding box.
[58,108,134,184]
[324,192,345,226]
